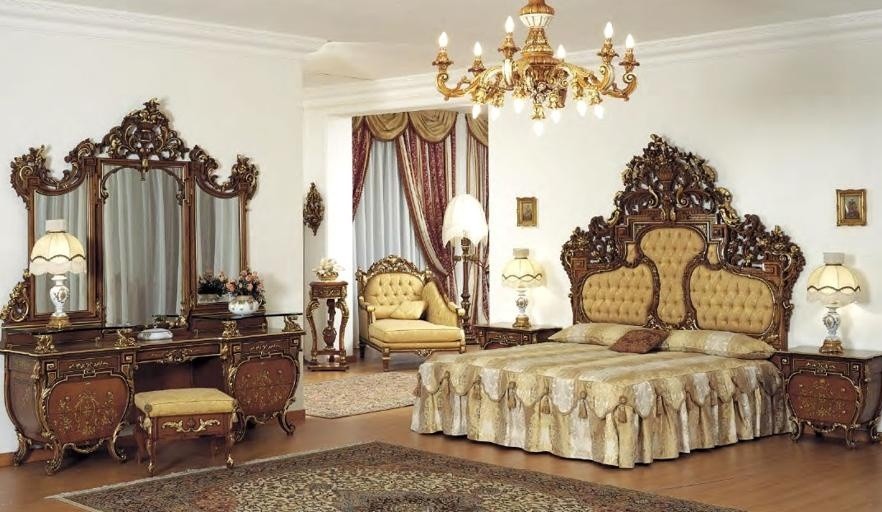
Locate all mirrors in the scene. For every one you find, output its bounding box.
[0,95,258,329]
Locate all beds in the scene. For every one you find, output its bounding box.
[408,134,804,467]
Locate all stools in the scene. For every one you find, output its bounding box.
[134,387,239,476]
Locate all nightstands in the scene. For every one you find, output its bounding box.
[474,321,562,350]
[778,345,882,448]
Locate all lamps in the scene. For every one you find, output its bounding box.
[805,252,861,352]
[29,218,88,328]
[500,247,546,327]
[441,194,488,344]
[432,0,640,119]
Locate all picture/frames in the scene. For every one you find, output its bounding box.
[835,188,867,226]
[516,196,537,227]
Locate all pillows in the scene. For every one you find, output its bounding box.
[390,300,428,320]
[548,323,777,359]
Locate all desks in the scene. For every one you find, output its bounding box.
[306,281,349,371]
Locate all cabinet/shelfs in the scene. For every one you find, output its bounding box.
[0,332,307,474]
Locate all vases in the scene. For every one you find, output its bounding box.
[317,270,338,281]
[228,296,258,314]
[197,293,228,303]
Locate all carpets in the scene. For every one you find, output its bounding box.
[304,371,415,419]
[45,440,752,512]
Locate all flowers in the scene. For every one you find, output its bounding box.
[311,256,335,274]
[196,272,229,295]
[223,265,264,299]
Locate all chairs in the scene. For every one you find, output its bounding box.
[357,254,466,372]
